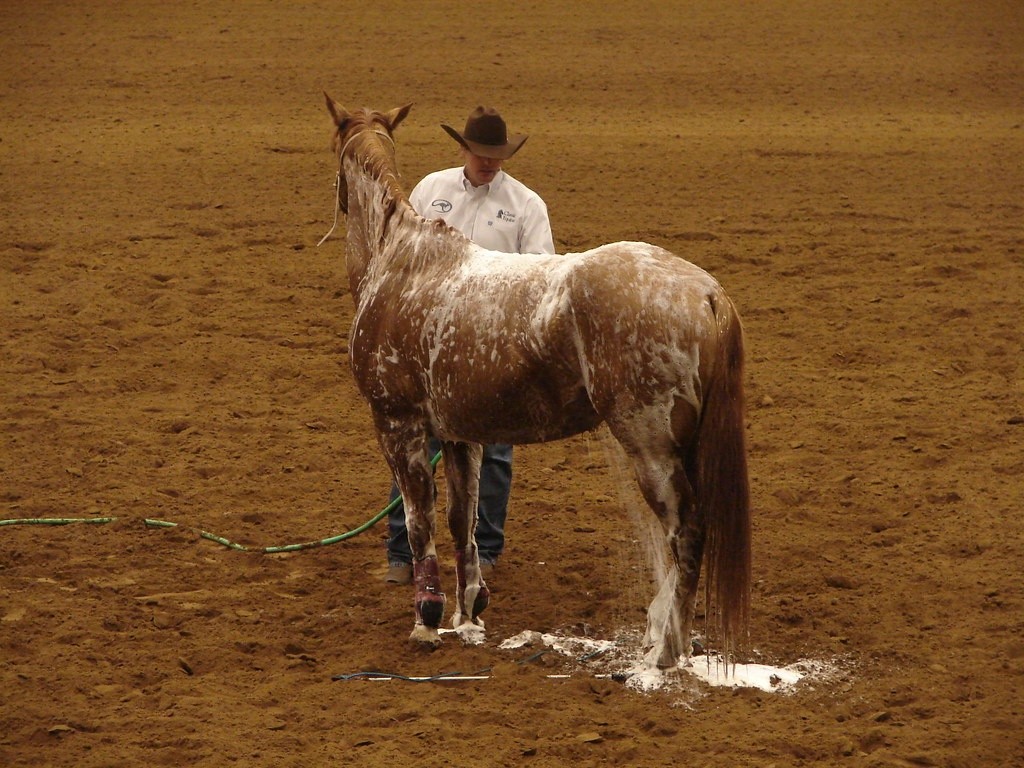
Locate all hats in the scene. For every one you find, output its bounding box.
[441,105,529,160]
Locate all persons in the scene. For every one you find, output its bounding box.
[384,106,557,587]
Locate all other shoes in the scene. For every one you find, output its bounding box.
[386,561,411,584]
[479,555,493,579]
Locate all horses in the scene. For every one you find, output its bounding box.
[318,89,749,672]
